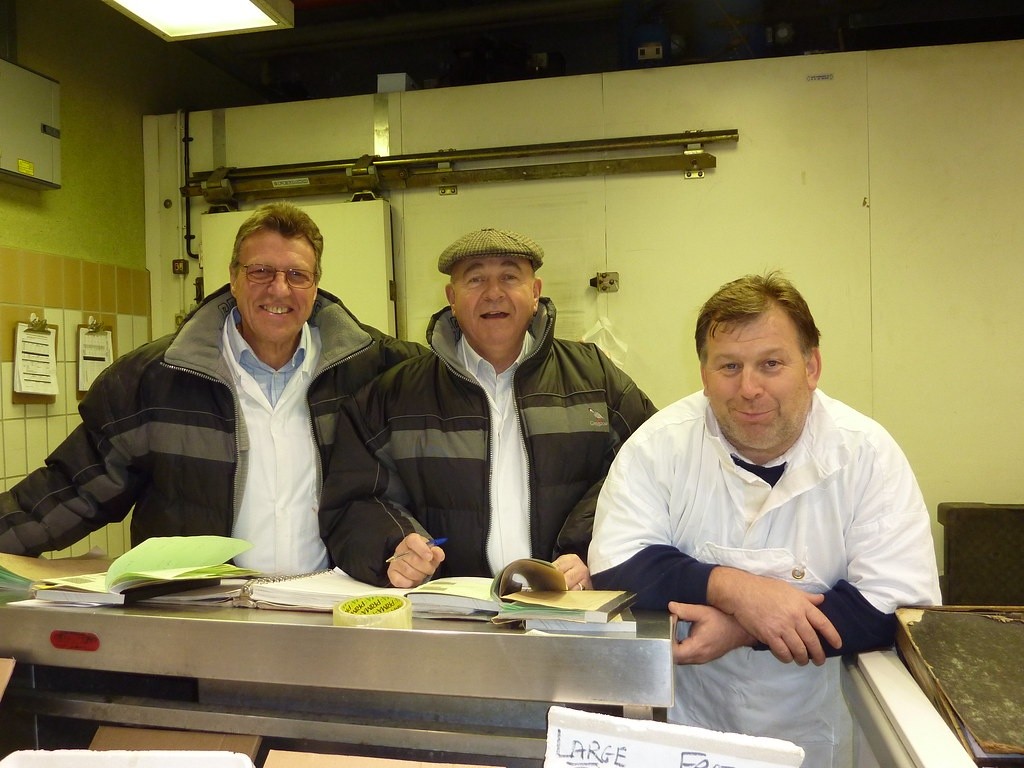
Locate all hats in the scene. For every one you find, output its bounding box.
[438,228,544,275]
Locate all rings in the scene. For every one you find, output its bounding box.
[577,583,585,591]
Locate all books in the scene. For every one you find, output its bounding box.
[0,536,637,632]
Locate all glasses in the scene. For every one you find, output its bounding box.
[235,263,320,288]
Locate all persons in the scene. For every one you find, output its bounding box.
[0,201,429,575]
[320,227,658,590]
[587,274,943,768]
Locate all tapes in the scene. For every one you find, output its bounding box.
[333,594,411,631]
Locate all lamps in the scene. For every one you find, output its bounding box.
[102,0,294,43]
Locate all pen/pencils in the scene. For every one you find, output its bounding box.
[385,538,448,562]
